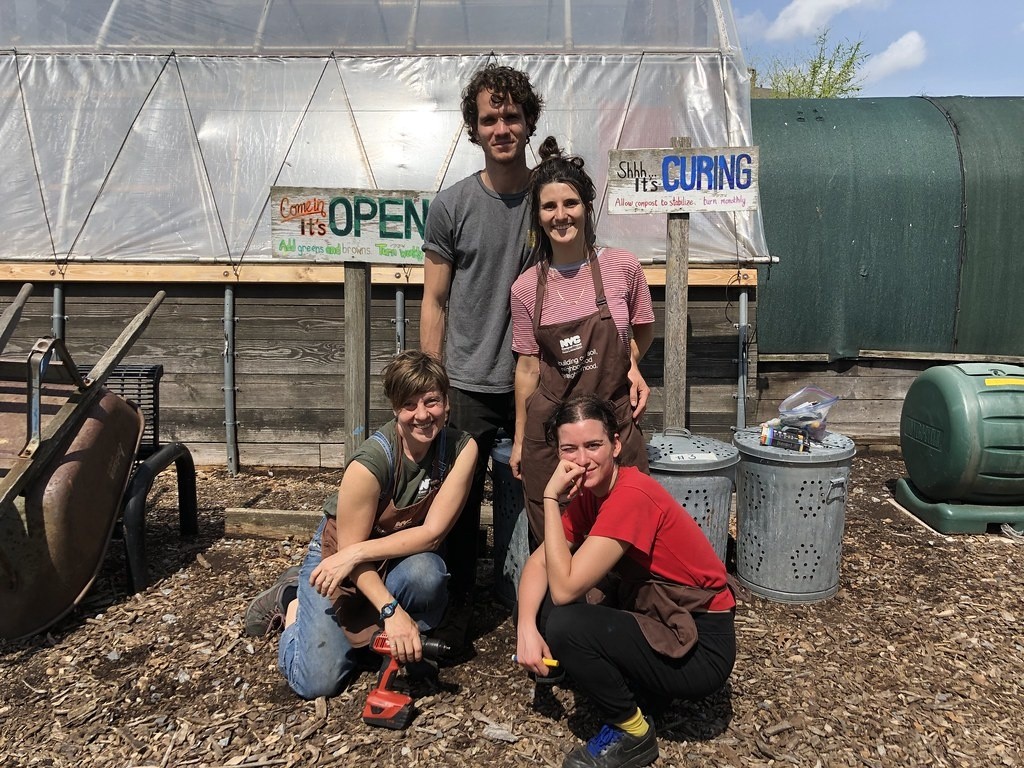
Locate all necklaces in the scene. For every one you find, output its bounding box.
[549,256,590,306]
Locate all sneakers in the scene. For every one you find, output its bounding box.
[560,713,659,768]
[246,565,301,639]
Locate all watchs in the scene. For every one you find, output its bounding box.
[379,599,398,620]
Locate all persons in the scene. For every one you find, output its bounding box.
[517,394,735,768]
[510,136,657,685]
[243,350,478,701]
[421,64,652,660]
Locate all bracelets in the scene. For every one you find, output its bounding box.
[543,496,560,503]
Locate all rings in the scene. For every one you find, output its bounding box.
[389,645,396,648]
[324,580,330,586]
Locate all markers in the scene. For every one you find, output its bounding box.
[511,654,560,667]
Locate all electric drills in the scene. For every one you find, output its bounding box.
[362,626,452,731]
